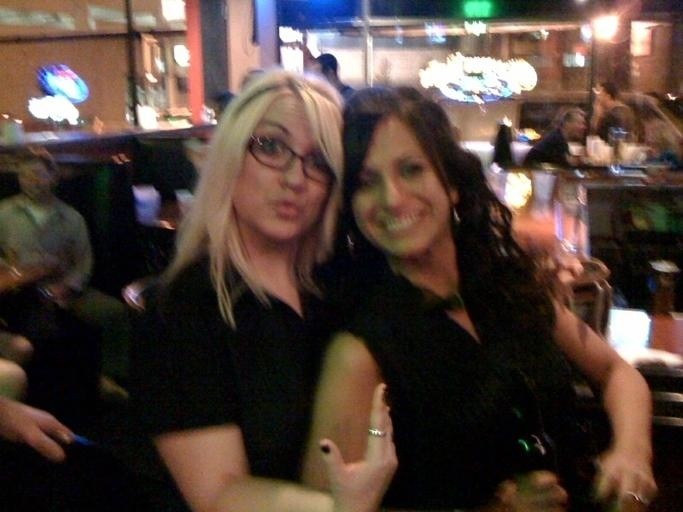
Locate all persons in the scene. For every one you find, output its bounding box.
[314,53,359,103]
[589,84,638,147]
[123,69,403,512]
[211,91,236,125]
[1,135,206,512]
[296,80,662,511]
[520,105,590,172]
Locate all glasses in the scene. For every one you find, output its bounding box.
[246,134,336,185]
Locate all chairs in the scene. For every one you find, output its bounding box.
[495,118,514,168]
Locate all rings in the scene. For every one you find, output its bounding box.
[369,428,386,438]
[632,493,644,504]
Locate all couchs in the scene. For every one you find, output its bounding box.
[1,157,136,289]
